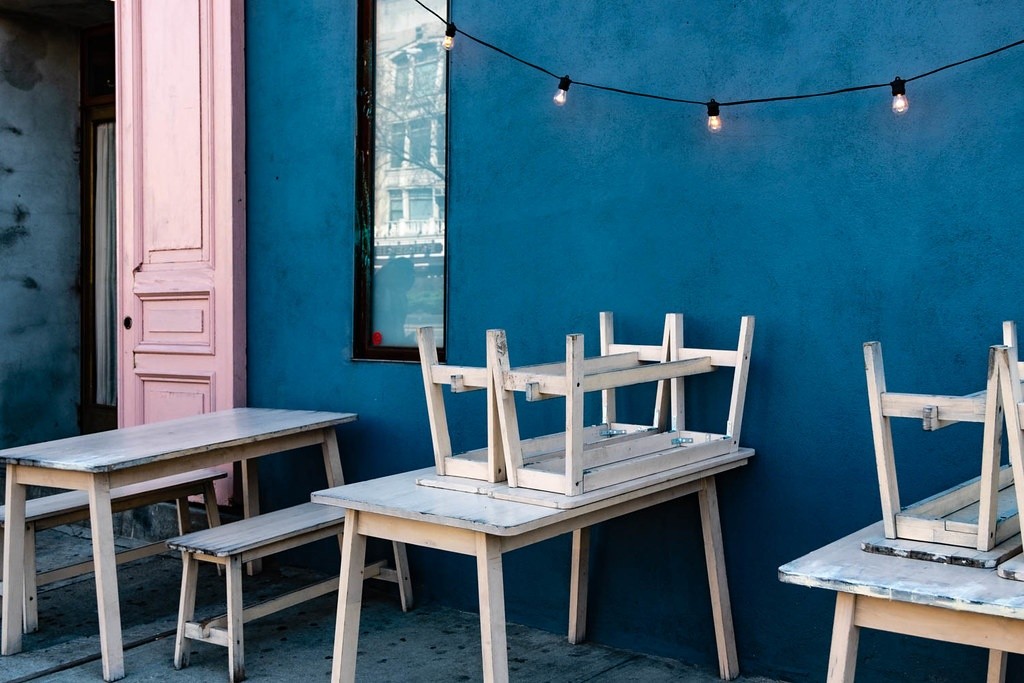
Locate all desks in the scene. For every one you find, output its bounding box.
[309,424,754,683]
[0,406,359,683]
[776,477,1024,683]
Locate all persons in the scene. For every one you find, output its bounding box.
[375,258,418,346]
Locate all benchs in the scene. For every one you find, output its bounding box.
[488,313,757,510]
[416,310,673,495]
[859,317,1024,582]
[0,467,229,635]
[165,501,415,683]
